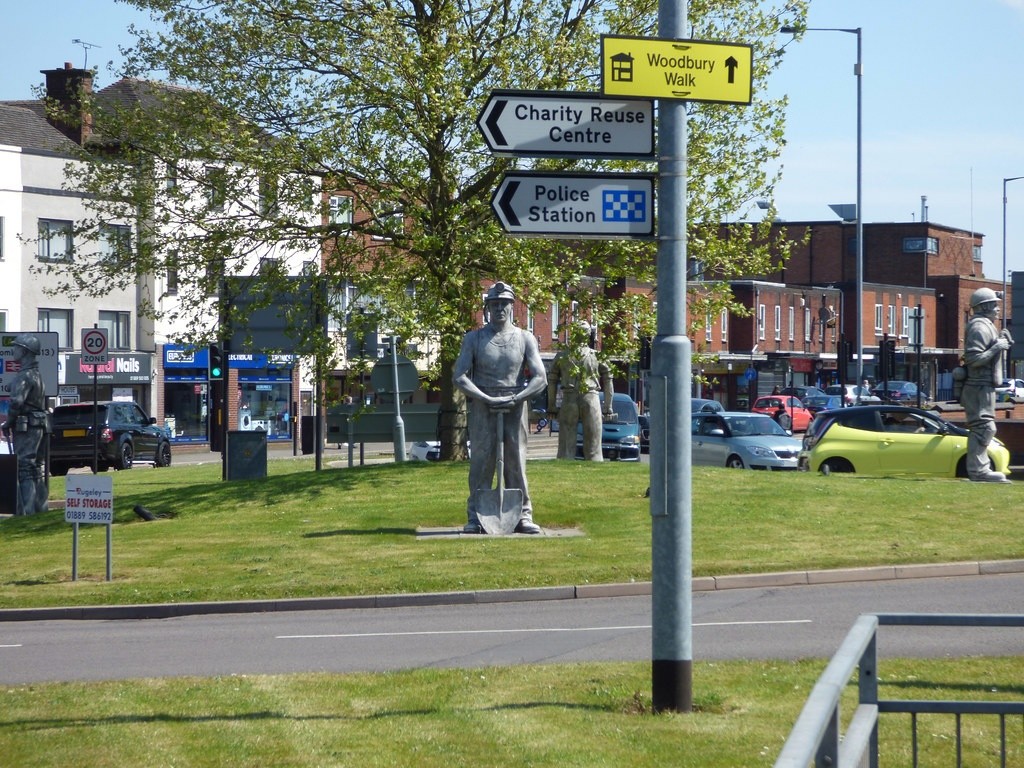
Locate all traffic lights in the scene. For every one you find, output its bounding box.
[209,343,223,378]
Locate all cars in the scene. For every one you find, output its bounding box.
[995,378,1024,404]
[575,392,642,462]
[800,395,854,418]
[408,441,472,460]
[871,381,928,401]
[798,401,1012,478]
[824,383,882,408]
[751,395,814,433]
[779,386,827,400]
[691,412,803,471]
[637,398,726,452]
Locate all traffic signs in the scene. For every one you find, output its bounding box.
[474,88,657,158]
[489,171,658,238]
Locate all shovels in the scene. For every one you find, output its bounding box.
[473,413,524,537]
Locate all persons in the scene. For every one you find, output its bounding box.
[339,396,357,449]
[961,287,1013,483]
[548,320,618,462]
[454,281,548,535]
[0,336,50,515]
[772,385,780,395]
[773,403,787,432]
[862,380,868,391]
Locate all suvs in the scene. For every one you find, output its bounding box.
[49,400,172,476]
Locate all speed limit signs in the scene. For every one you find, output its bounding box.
[82,329,108,364]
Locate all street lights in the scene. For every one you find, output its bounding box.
[781,26,863,400]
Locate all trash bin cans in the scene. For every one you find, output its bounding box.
[302,416,317,454]
